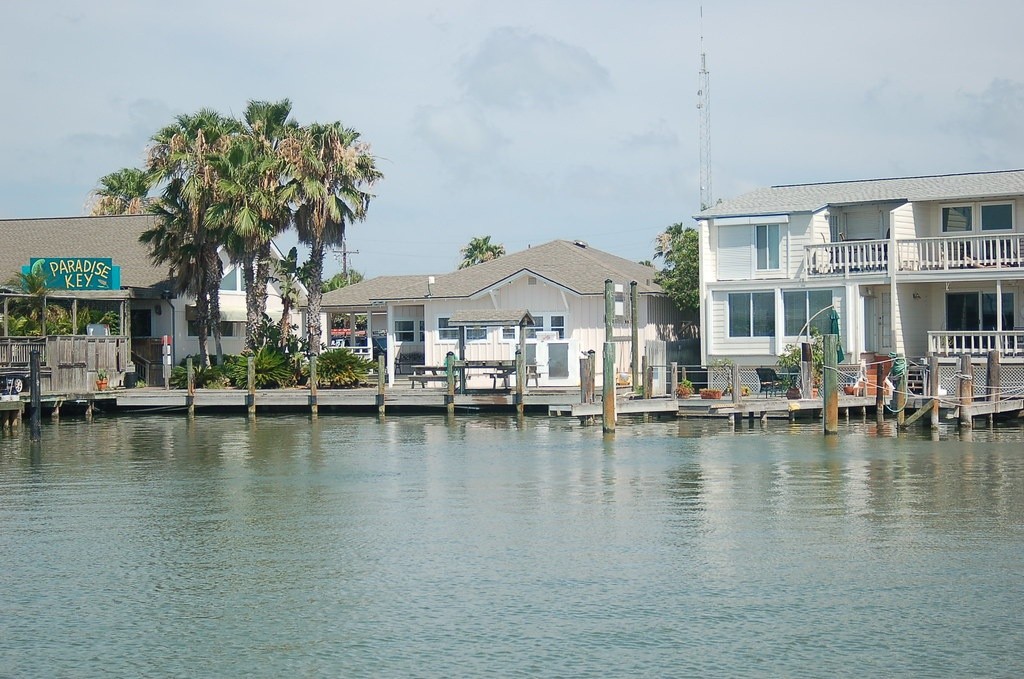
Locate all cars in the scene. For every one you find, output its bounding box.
[332,335,387,363]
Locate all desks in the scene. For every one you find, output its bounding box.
[842,238,876,270]
[410,365,457,390]
[497,366,538,388]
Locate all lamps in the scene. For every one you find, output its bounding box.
[913,293,921,300]
[867,289,871,294]
[424,276,435,298]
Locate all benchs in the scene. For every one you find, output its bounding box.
[394,353,425,375]
[483,372,547,378]
[407,375,471,381]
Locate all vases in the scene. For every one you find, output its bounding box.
[843,386,854,395]
[698,389,722,399]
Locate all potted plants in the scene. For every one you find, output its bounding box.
[120,372,138,388]
[674,377,695,399]
[96,368,109,390]
[774,325,825,399]
[728,387,750,396]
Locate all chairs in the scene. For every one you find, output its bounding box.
[821,232,845,273]
[992,327,1011,356]
[871,228,890,270]
[1013,327,1024,356]
[755,367,786,399]
